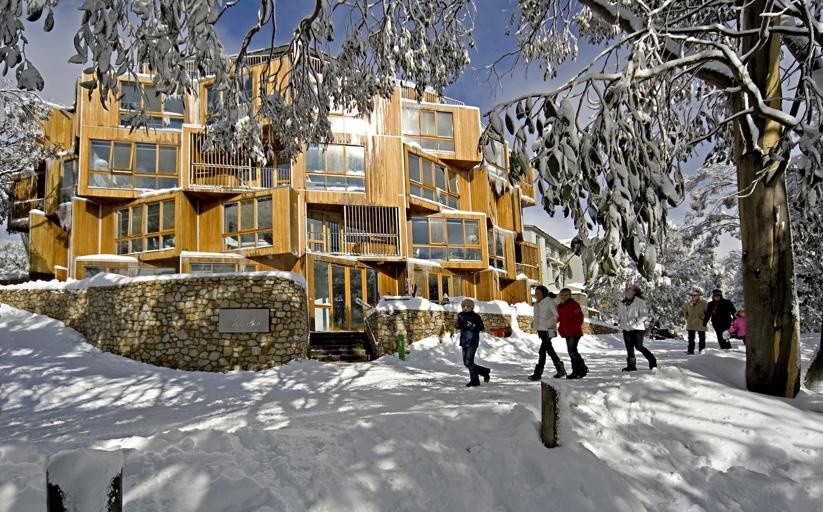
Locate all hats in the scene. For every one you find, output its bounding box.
[713,289,722,297]
[690,291,699,295]
[461,299,474,309]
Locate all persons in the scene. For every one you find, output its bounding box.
[618,284,658,372]
[527,285,567,381]
[684,291,708,355]
[728,307,746,345]
[556,287,590,380]
[702,289,737,349]
[454,298,491,387]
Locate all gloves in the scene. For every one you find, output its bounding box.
[465,321,475,330]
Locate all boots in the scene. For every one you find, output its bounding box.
[646,354,657,370]
[622,358,636,372]
[466,366,490,387]
[529,354,588,380]
[687,346,694,355]
